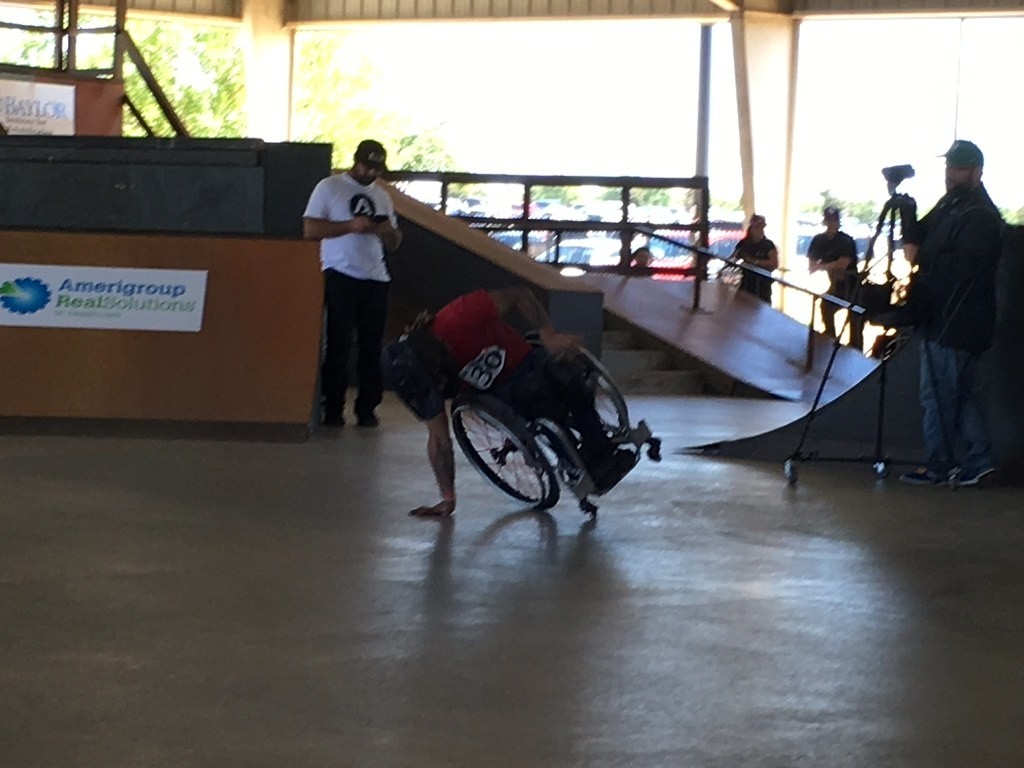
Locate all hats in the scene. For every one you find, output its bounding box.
[750,216,766,226]
[378,333,444,421]
[937,140,984,170]
[354,140,389,174]
[824,207,840,219]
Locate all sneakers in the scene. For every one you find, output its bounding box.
[899,462,961,485]
[953,457,995,488]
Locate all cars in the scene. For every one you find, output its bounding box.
[410,193,745,279]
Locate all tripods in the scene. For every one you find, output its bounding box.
[785,183,963,489]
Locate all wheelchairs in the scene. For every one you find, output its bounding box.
[449,328,661,517]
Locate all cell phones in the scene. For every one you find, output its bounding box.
[371,215,389,224]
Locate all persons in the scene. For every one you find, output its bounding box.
[631,247,653,267]
[302,140,399,427]
[899,140,1000,484]
[731,215,778,306]
[371,285,616,518]
[808,206,863,353]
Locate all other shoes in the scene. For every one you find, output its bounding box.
[358,411,377,427]
[324,413,345,426]
[590,447,635,489]
